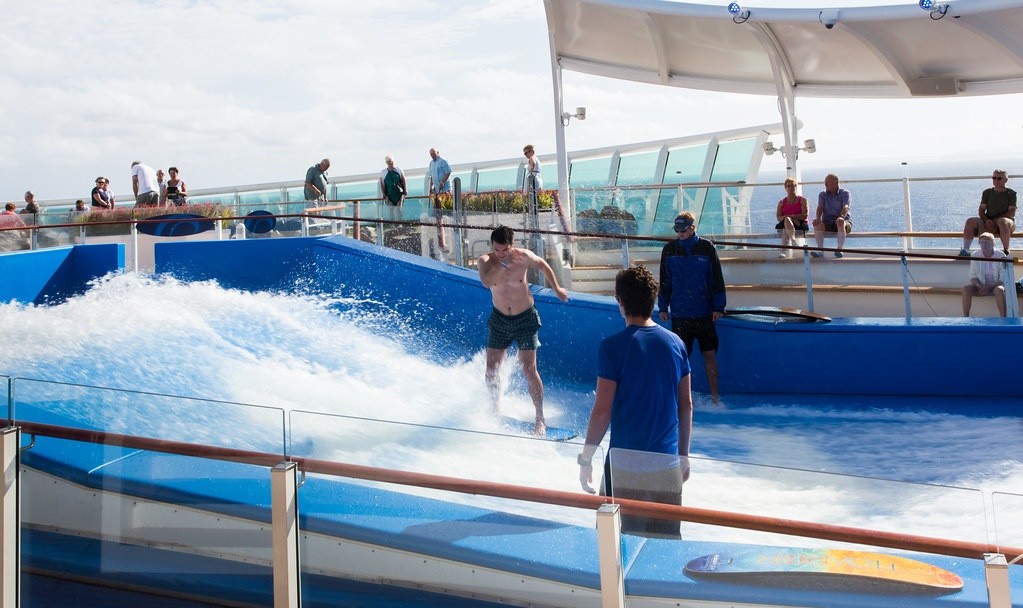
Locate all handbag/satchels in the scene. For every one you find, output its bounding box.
[395,184,404,194]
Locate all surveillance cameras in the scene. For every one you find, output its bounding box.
[821,9,839,29]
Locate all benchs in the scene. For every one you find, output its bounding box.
[634,231,1023,321]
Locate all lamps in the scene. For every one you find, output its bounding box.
[564,107,586,120]
[762,141,786,159]
[795,139,815,160]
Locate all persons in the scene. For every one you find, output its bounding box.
[20,190,48,235]
[957,168,1018,256]
[523,144,543,189]
[71,199,90,219]
[91,176,115,209]
[810,173,853,258]
[658,211,726,404]
[580,266,693,539]
[961,231,1006,318]
[156,170,167,206]
[774,177,810,259]
[162,166,188,206]
[428,147,453,216]
[131,161,160,208]
[303,159,330,215]
[380,155,408,219]
[0,201,29,237]
[477,224,568,436]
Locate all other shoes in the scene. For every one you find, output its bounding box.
[1004,250,1011,256]
[810,252,823,257]
[834,252,843,257]
[958,250,971,257]
[779,254,785,258]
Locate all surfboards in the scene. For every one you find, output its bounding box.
[493,415,580,442]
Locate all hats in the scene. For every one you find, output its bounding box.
[671,220,692,229]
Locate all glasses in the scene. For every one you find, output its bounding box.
[786,185,795,188]
[992,176,1004,180]
[524,151,531,154]
[673,227,690,233]
[97,182,104,184]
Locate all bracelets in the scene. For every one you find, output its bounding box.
[577,454,592,466]
[502,262,510,269]
[839,215,845,219]
